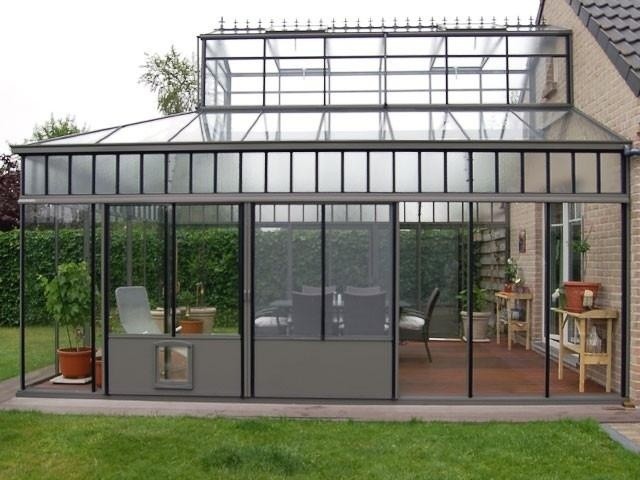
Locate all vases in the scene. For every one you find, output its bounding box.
[456,284,492,338]
[150,310,165,331]
[504,284,528,294]
[89,357,102,388]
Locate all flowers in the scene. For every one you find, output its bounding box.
[504,258,522,285]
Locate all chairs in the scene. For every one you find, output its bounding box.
[290,292,334,338]
[398,288,440,362]
[255,308,287,337]
[346,285,382,294]
[303,285,337,303]
[116,286,187,370]
[342,292,385,336]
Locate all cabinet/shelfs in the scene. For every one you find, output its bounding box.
[494,292,532,352]
[471,227,510,314]
[551,308,618,393]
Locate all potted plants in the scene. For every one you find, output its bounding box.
[564,217,601,313]
[35,260,100,379]
[177,291,203,333]
[188,283,217,335]
